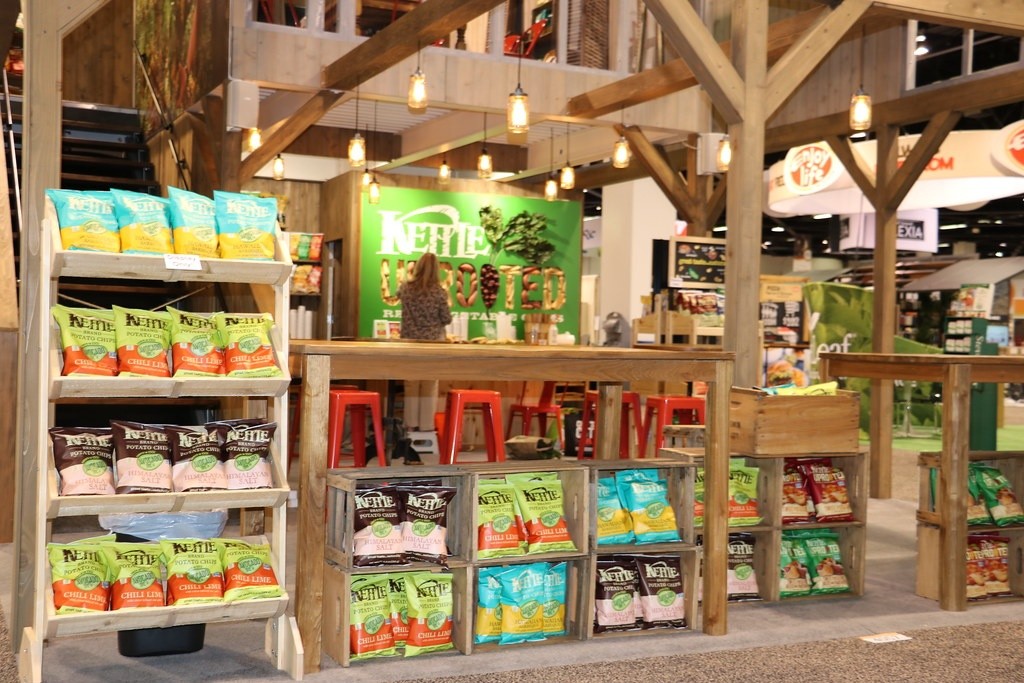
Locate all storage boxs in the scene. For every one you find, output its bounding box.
[728,384,863,457]
[448,458,590,562]
[777,524,868,603]
[688,528,776,609]
[318,560,472,656]
[663,426,704,449]
[322,471,475,571]
[570,455,699,550]
[780,452,873,529]
[584,546,705,639]
[913,523,1024,608]
[473,557,589,650]
[909,450,1024,531]
[659,446,777,531]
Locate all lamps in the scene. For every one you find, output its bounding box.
[271,151,287,182]
[560,123,575,191]
[542,130,557,200]
[714,133,732,172]
[408,40,431,111]
[477,111,493,180]
[438,151,452,185]
[851,25,872,131]
[368,99,381,204]
[362,122,371,184]
[612,132,629,171]
[349,88,367,167]
[507,28,531,134]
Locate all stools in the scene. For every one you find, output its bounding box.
[577,389,642,457]
[328,387,389,467]
[645,392,706,456]
[440,387,506,464]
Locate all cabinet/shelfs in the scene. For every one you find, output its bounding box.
[287,219,339,351]
[12,181,307,683]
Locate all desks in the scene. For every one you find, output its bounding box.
[289,338,737,635]
[819,347,1024,611]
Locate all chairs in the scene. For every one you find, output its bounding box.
[505,379,571,450]
